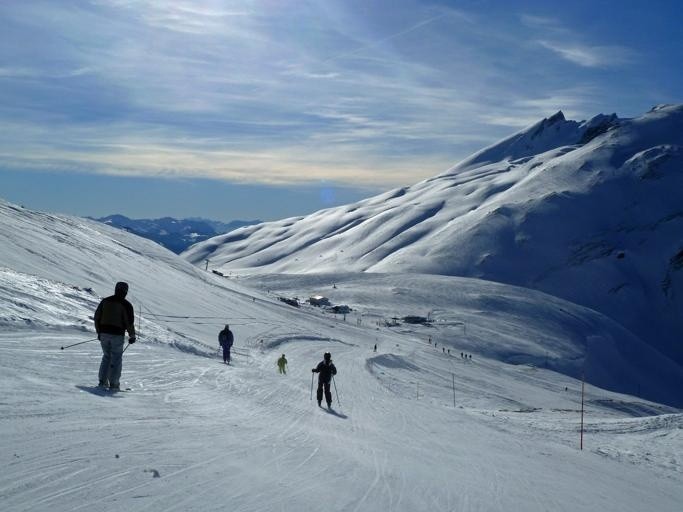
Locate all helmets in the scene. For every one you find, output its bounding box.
[324,352,331,358]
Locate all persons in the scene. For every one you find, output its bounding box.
[218,324,234,364]
[253,297,257,302]
[312,352,337,408]
[429,337,471,360]
[94,282,136,390]
[278,354,287,375]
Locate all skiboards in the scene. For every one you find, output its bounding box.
[93,385,132,393]
[220,361,233,366]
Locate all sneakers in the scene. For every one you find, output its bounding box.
[99,379,120,390]
[318,399,331,408]
